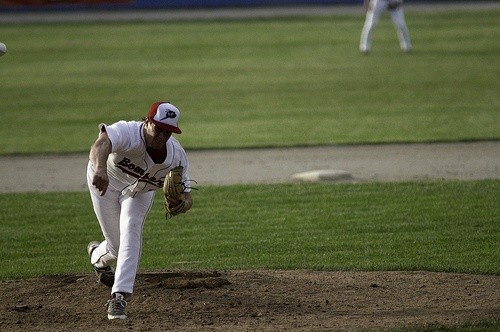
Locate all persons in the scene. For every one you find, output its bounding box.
[86,101,195,320]
[359,0,412,55]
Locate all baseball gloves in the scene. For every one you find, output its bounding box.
[162,165,186,217]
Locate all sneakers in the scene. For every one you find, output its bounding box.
[87,241,115,287]
[105,293,127,320]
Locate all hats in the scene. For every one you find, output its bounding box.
[148,102,181,134]
